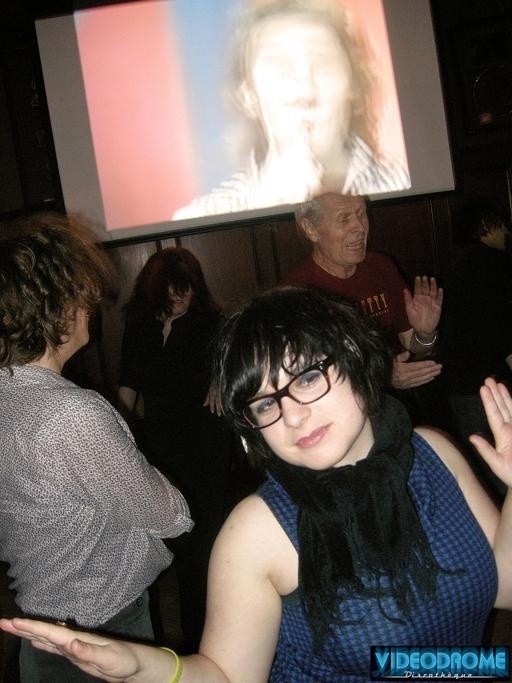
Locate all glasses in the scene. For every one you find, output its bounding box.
[242,347,348,429]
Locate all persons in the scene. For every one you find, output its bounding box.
[0,285,512,683]
[279,173,445,428]
[169,4,411,224]
[0,207,196,682]
[120,246,231,643]
[430,192,512,503]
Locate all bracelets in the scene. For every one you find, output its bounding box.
[414,330,440,348]
[159,646,183,682]
